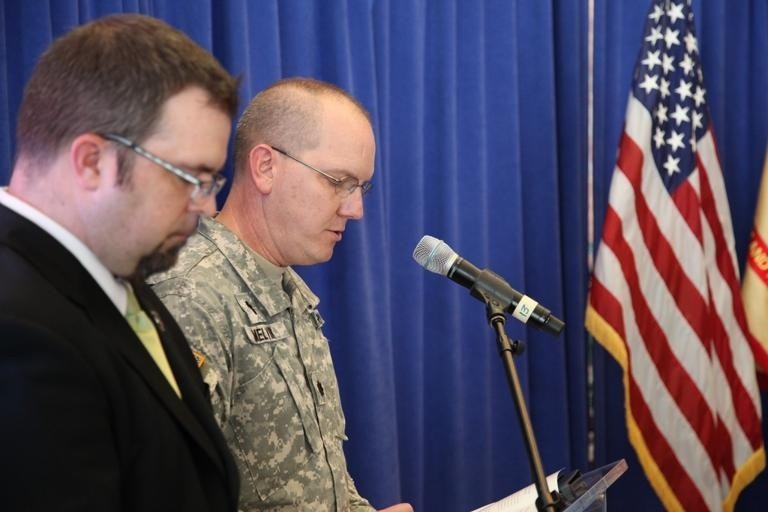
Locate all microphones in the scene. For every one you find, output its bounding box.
[411,233,566,339]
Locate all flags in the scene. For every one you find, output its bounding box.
[582,0,767,512]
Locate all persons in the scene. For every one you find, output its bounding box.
[0,14,243,512]
[144,75,414,512]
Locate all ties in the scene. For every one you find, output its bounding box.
[115,276,181,402]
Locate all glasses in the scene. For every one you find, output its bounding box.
[103,132,227,205]
[271,145,373,197]
